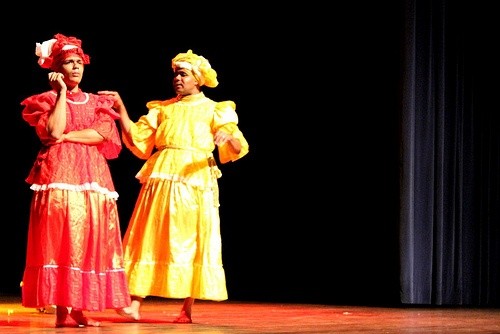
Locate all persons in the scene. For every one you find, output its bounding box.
[20,33,132,328]
[97,49,250,323]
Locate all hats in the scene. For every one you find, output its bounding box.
[172,50,218,88]
[35,33,90,71]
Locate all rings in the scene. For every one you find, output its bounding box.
[221,135,226,140]
